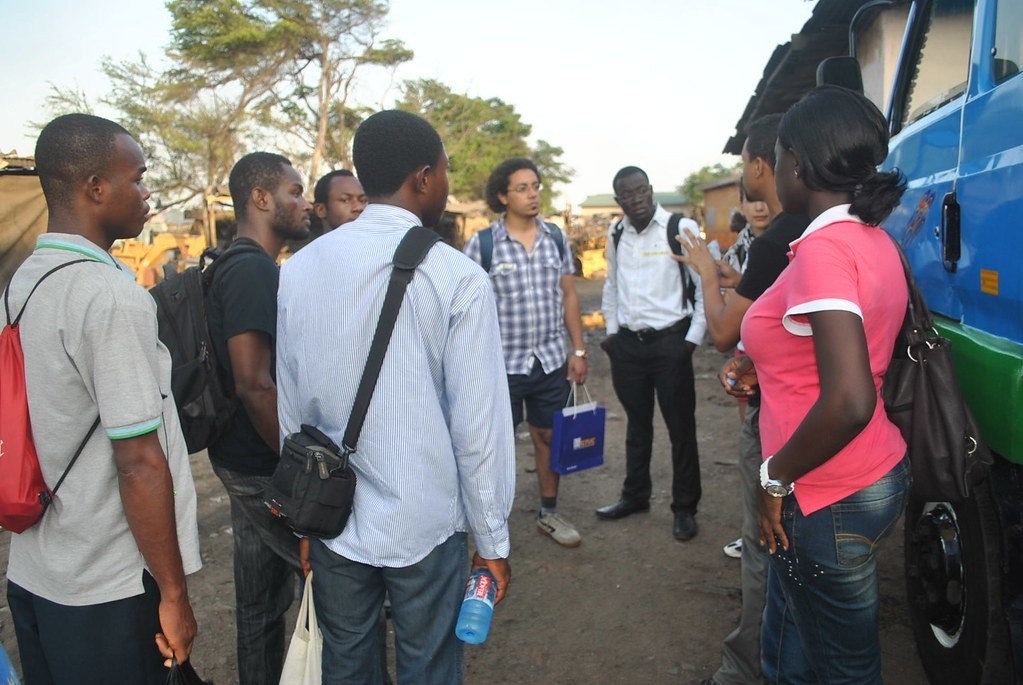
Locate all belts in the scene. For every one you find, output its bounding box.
[620,319,691,342]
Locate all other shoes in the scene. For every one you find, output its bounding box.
[724,538,745,560]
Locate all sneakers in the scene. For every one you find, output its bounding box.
[537,511,584,547]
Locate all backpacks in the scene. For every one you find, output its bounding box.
[148,242,263,453]
[0,258,101,533]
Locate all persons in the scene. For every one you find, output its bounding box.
[0,113,204,685]
[595,167,708,542]
[200,150,314,685]
[459,157,588,549]
[671,84,909,685]
[313,169,369,234]
[275,109,517,685]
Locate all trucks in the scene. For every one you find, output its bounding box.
[813,0,1023,685]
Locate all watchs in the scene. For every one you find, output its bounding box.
[573,349,588,358]
[760,455,795,498]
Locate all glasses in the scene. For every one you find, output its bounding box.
[506,183,543,195]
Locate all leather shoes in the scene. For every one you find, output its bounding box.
[673,514,698,538]
[594,499,648,519]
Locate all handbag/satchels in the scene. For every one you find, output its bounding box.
[277,569,323,685]
[167,656,204,685]
[881,227,993,503]
[551,381,606,475]
[261,422,363,537]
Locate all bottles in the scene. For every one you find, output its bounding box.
[455,566,498,645]
